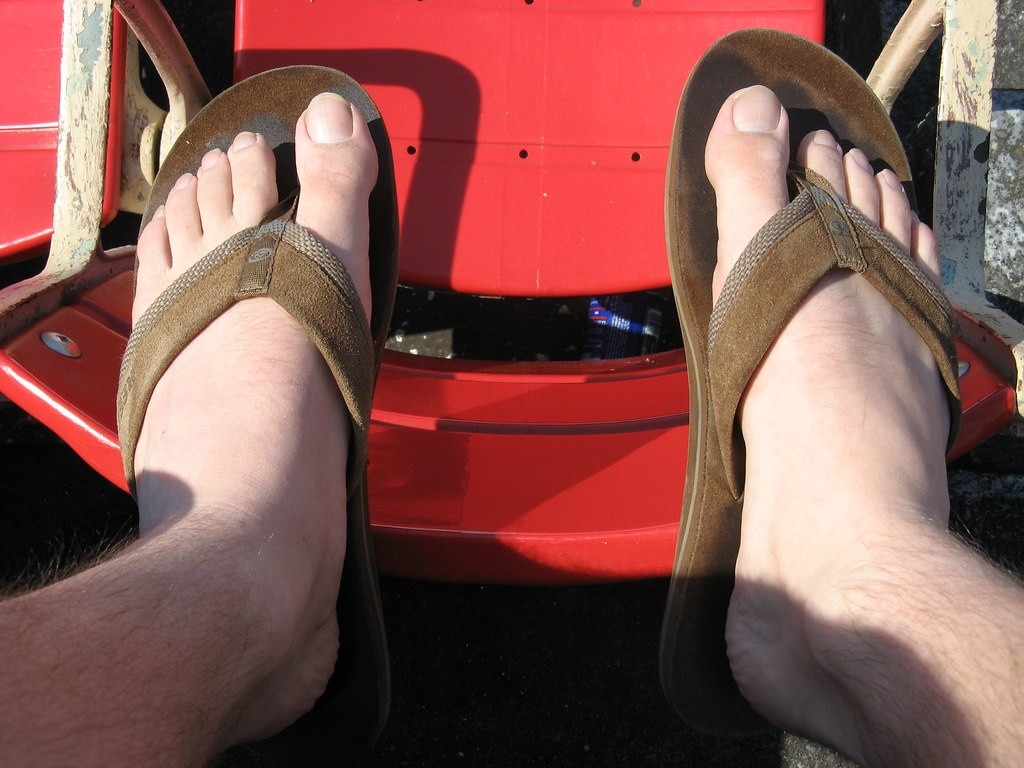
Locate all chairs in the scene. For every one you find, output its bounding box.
[0,0,1024,582]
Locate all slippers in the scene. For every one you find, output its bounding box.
[116,64,390,768]
[657,25,963,739]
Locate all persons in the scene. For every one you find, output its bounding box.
[0,28,1024,768]
